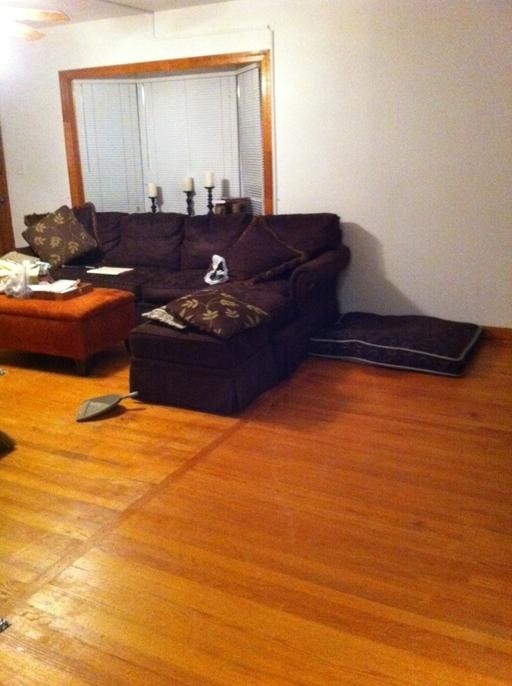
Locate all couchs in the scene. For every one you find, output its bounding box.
[14,203,351,416]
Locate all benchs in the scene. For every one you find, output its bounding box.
[0,282,137,376]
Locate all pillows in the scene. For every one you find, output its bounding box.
[167,282,291,338]
[23,204,95,267]
[27,204,103,245]
[218,213,308,281]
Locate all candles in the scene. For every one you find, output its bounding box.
[205,171,215,186]
[148,183,158,196]
[184,176,194,190]
